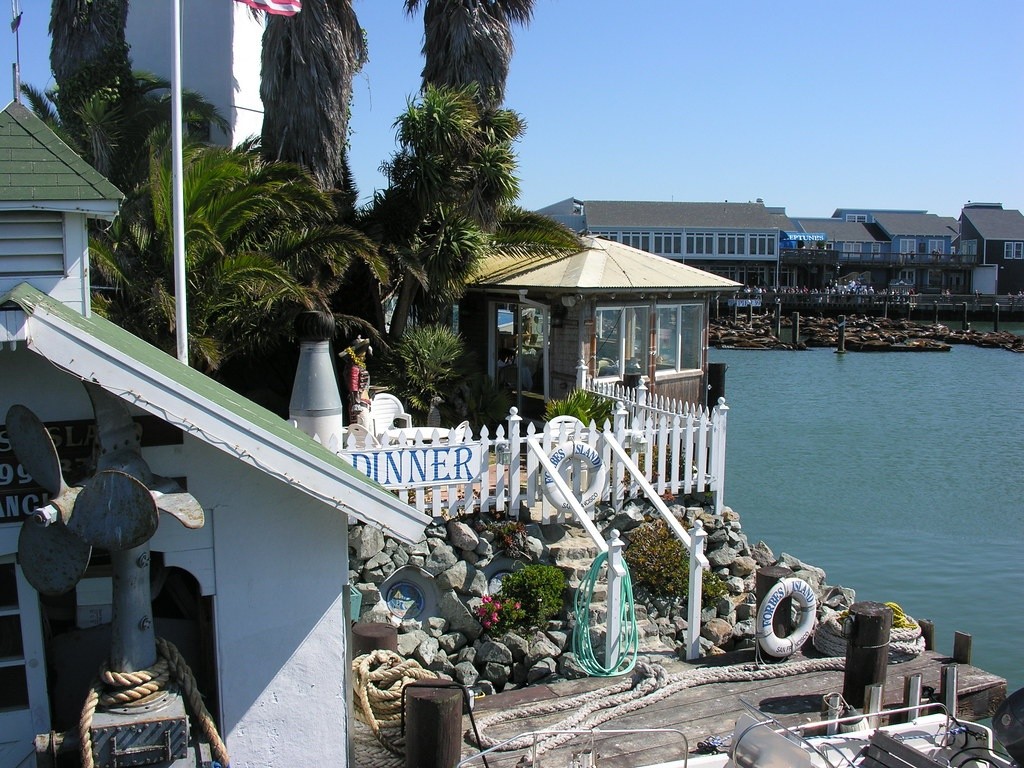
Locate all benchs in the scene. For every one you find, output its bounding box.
[863,731,949,768]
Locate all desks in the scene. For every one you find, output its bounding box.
[383,427,451,446]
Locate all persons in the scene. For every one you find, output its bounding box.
[744,284,1024,295]
[339,335,371,424]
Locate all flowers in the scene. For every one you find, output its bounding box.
[346,346,367,369]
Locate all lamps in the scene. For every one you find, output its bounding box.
[495,443,512,465]
[633,433,648,454]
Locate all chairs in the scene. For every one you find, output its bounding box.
[346,424,381,450]
[548,416,588,489]
[452,420,472,445]
[596,357,615,377]
[369,393,412,445]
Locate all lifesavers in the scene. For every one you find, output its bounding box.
[540,440,607,513]
[754,574,820,659]
